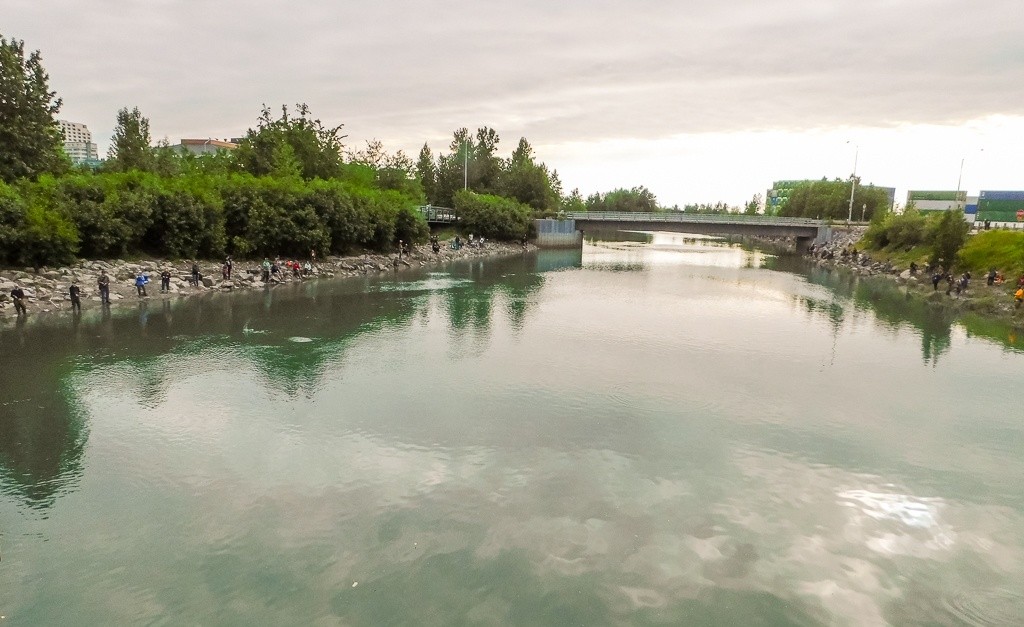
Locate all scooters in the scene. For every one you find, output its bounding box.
[429,234,440,253]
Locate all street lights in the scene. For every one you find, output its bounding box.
[846,140,859,227]
[955,148,985,211]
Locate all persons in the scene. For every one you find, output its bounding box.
[98,270,112,304]
[11,283,26,315]
[161,268,170,290]
[222,255,232,280]
[984,219,990,230]
[430,235,440,252]
[399,240,410,259]
[69,281,81,310]
[456,232,486,250]
[136,270,148,296]
[807,244,1024,308]
[293,250,316,277]
[262,258,279,282]
[522,233,528,246]
[192,261,199,286]
